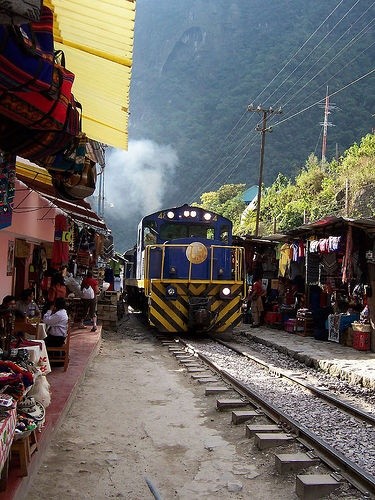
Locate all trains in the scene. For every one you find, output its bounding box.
[123,203,247,342]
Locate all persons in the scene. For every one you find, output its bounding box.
[248,272,304,328]
[0,263,121,366]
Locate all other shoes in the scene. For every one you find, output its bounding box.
[78,325,87,329]
[91,326,97,331]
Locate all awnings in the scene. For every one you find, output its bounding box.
[15,0,136,235]
[241,215,375,244]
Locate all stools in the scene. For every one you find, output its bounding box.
[10,429,39,476]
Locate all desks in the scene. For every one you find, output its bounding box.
[18,340,52,375]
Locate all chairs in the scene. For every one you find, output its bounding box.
[46,327,70,371]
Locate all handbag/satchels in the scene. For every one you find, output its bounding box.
[81,285,95,300]
